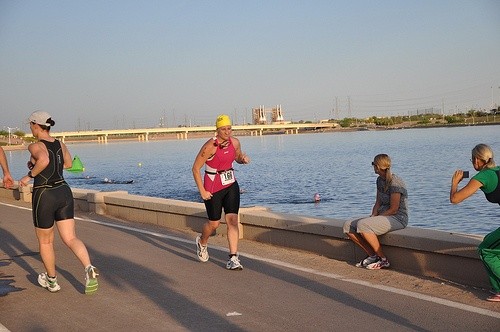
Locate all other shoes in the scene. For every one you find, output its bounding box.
[487,291,499,303]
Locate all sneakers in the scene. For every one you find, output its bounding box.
[366,258,391,270]
[195,236,210,262]
[82,264,99,296]
[355,256,379,268]
[225,254,243,271]
[36,271,60,294]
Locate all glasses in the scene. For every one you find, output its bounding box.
[371,161,379,166]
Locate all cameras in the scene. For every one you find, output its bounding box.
[462,171,469,178]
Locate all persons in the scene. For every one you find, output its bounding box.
[342,153,409,271]
[450,144,500,302]
[191,115,249,271]
[0,146,14,189]
[19,110,100,296]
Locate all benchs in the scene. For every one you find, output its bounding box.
[0,180,34,202]
[104,194,271,240]
[30,186,128,215]
[240,211,493,289]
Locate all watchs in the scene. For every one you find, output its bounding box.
[28,170,35,178]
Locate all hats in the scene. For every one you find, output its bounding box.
[217,114,231,129]
[23,109,54,128]
